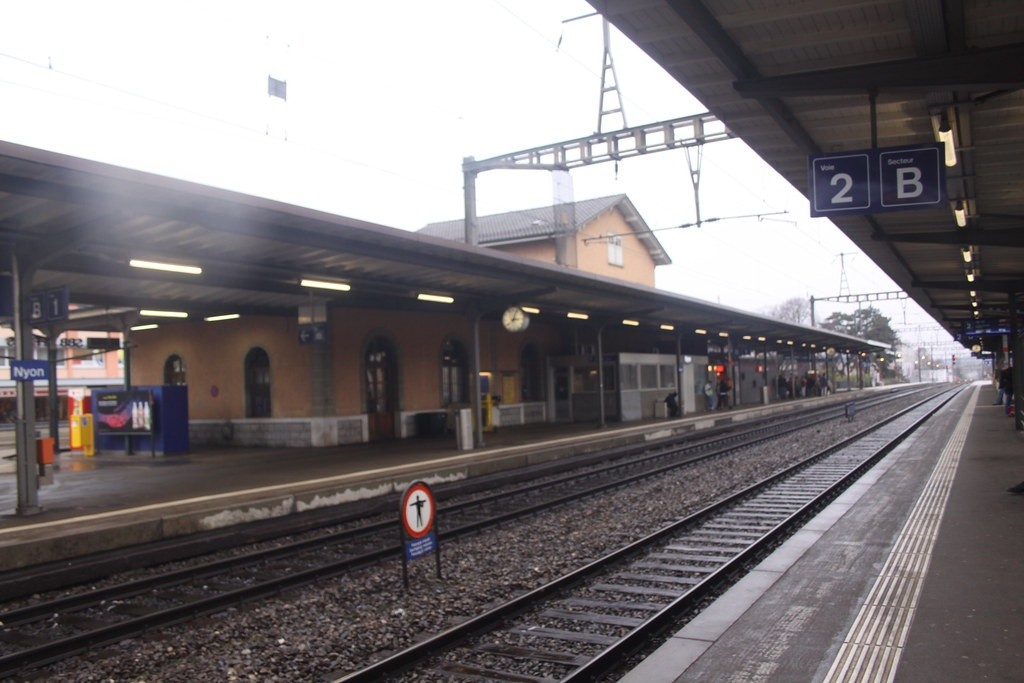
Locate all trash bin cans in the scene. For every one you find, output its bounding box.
[760,386,770,405]
[654,398,670,418]
[454,409,475,450]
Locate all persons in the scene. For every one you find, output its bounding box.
[800,375,807,397]
[820,373,827,396]
[779,374,792,399]
[1003,366,1012,414]
[664,392,678,417]
[716,380,732,409]
[705,380,715,410]
[795,374,801,398]
[992,369,1004,405]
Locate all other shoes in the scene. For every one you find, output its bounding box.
[1006,481,1024,492]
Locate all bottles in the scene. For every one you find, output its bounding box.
[132,401,151,431]
[70,403,81,449]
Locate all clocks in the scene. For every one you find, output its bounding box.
[503,306,529,333]
[972,344,982,353]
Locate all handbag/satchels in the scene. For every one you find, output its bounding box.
[720,392,727,396]
[1008,404,1015,416]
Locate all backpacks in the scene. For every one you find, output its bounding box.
[1003,367,1012,385]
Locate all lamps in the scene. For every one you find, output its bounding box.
[622,319,639,326]
[129,323,158,331]
[963,247,972,263]
[967,269,974,282]
[566,312,589,320]
[137,307,190,318]
[298,277,350,292]
[970,289,976,297]
[415,293,453,303]
[936,115,957,168]
[521,307,540,314]
[954,200,966,228]
[128,259,202,275]
[660,325,674,330]
[202,314,241,321]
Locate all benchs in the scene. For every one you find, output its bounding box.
[654,396,679,418]
